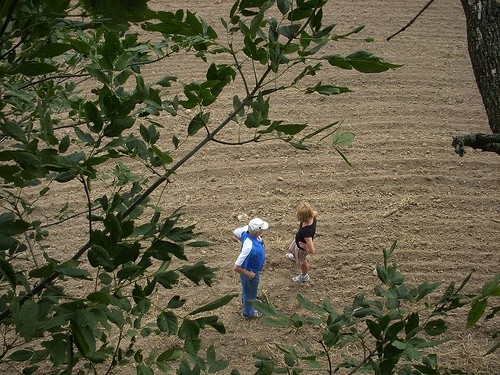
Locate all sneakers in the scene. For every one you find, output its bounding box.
[291,273,310,282]
[285,252,295,260]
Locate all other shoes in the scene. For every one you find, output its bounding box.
[242,310,261,318]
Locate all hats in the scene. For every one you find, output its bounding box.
[247,217,269,234]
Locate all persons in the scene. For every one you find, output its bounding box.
[285,204,318,281]
[233,218,269,319]
